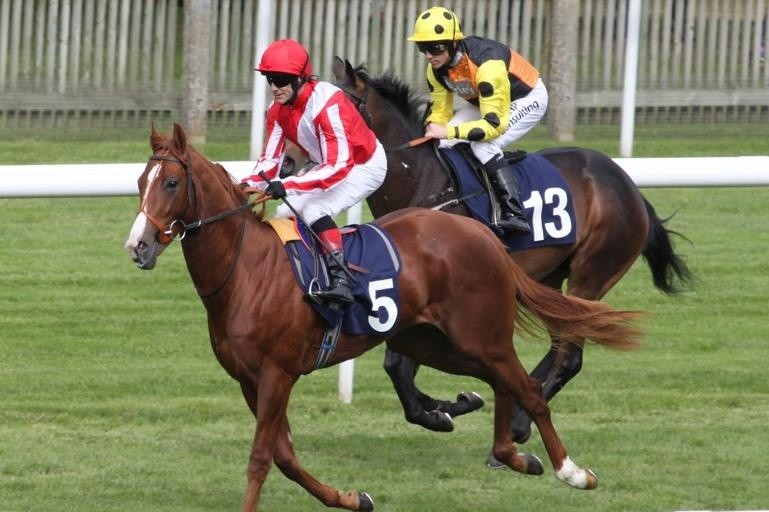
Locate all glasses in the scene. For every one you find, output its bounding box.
[267,74,293,89]
[416,41,447,55]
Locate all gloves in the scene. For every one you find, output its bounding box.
[265,182,286,199]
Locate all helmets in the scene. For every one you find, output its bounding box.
[407,6,466,41]
[253,39,311,80]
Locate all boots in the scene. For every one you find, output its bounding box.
[303,228,370,304]
[491,166,532,235]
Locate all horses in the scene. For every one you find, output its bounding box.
[331,54,701,467]
[124,122,660,512]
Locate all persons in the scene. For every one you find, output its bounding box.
[237,38,390,302]
[408,7,548,233]
